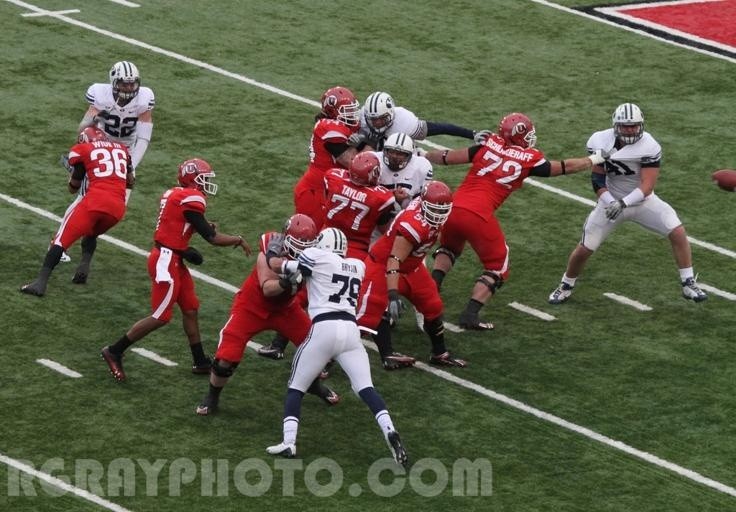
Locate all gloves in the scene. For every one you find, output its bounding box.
[268,231,285,256]
[605,199,626,221]
[589,149,609,165]
[93,110,109,123]
[386,289,408,328]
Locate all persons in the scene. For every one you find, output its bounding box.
[417,112,610,331]
[21,127,137,296]
[548,102,708,303]
[193,83,494,470]
[47,61,155,263]
[103,158,253,381]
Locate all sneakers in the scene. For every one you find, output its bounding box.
[20,283,44,296]
[191,304,494,466]
[47,245,71,262]
[681,277,708,302]
[72,270,86,284]
[548,282,574,304]
[100,346,126,383]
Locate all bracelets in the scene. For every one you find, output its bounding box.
[622,187,645,207]
[599,191,614,207]
[233,235,242,249]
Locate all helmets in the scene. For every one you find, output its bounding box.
[612,103,644,145]
[110,61,140,102]
[78,126,108,143]
[283,86,537,260]
[179,159,217,196]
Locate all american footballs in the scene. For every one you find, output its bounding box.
[712,169,736,191]
